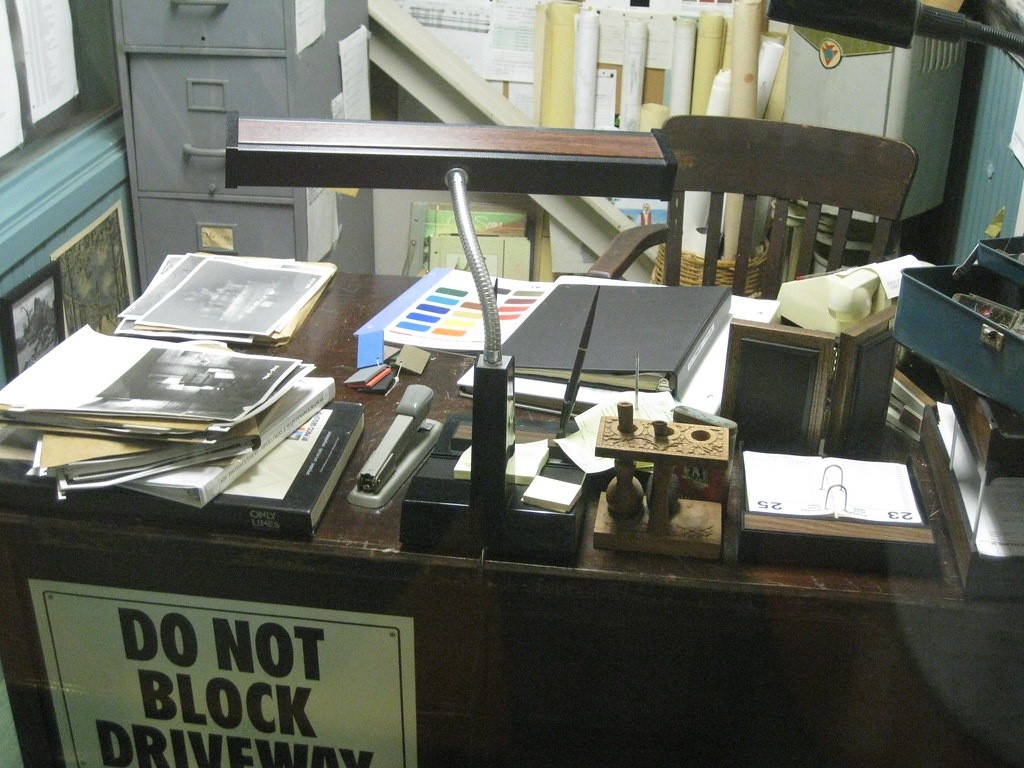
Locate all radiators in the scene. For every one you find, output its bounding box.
[940,8,1023,265]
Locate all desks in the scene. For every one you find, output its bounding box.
[1,275,1024,768]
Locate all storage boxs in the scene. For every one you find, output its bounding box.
[892,236,1024,420]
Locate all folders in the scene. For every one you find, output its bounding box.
[456,274,781,423]
[354,266,557,360]
[118,373,337,511]
[500,280,734,403]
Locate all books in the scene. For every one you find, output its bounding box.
[457,272,785,422]
[453,437,550,484]
[0,320,368,541]
[521,477,583,513]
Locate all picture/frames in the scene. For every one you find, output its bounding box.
[1,261,66,383]
[718,302,899,457]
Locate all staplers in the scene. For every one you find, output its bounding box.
[348,384,445,512]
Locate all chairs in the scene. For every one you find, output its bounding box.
[590,114,918,300]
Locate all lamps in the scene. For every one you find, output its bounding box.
[225,114,678,569]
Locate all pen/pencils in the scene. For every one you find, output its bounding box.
[555,285,604,439]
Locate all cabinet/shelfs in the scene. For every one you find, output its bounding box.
[112,1,373,296]
